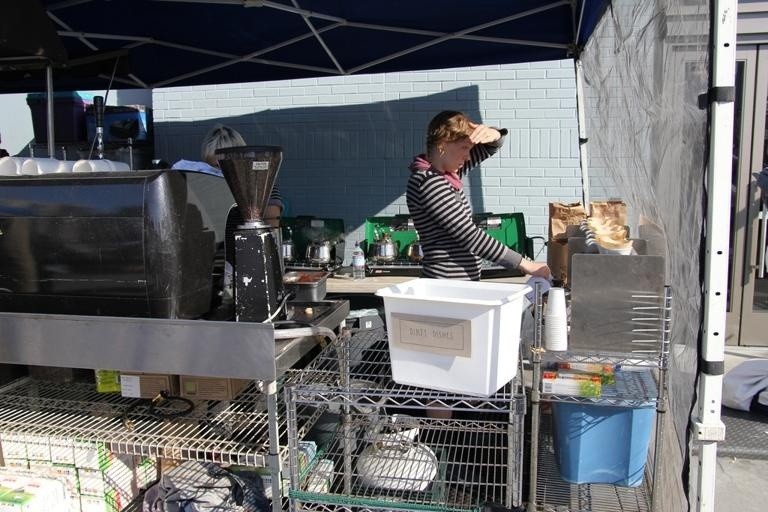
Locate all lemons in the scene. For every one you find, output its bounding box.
[283,226,337,264]
[369,232,425,262]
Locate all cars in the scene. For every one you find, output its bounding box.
[0,156,132,177]
[543,287,568,361]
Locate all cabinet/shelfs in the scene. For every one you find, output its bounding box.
[351,243,367,281]
[0,282,673,512]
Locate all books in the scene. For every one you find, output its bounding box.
[0,433,159,512]
[94,369,121,392]
[375,278,533,399]
[180,375,252,402]
[549,396,655,487]
[120,372,180,399]
[29,364,90,383]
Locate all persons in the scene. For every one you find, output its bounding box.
[405,109,552,490]
[200,123,285,229]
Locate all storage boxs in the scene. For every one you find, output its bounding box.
[29,364,90,383]
[120,372,180,399]
[549,396,655,487]
[0,433,159,512]
[94,369,121,392]
[375,278,533,399]
[180,375,252,402]
